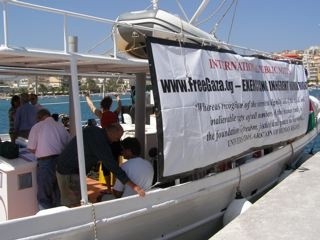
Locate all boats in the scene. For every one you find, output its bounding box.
[0,0,320,240]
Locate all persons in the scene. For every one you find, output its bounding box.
[83,89,124,194]
[148,148,157,185]
[97,137,154,201]
[27,109,72,210]
[9,93,70,142]
[56,123,146,208]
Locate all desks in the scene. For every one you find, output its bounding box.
[0,160,38,220]
